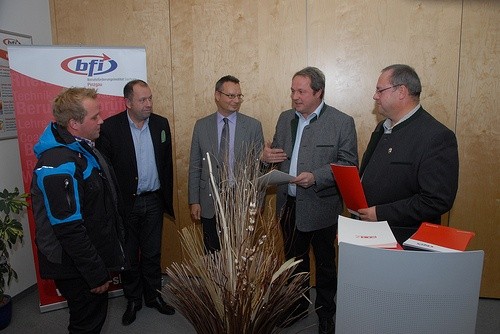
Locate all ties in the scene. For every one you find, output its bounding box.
[219,118,230,190]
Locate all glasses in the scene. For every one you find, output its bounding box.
[376,84,404,94]
[216,89,244,99]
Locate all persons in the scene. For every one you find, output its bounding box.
[351,65,459,251]
[29,86,128,334]
[258,67,359,334]
[94,79,176,326]
[188,74,267,256]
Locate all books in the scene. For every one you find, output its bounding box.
[250,169,295,185]
[386,241,404,250]
[337,214,397,249]
[403,222,475,252]
[330,163,368,217]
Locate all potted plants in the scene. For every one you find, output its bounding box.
[0,186,32,331]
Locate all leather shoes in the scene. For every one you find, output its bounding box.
[277,309,311,327]
[121,296,142,326]
[318,322,335,334]
[144,295,176,316]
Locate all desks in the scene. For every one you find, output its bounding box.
[334,238,485,333]
